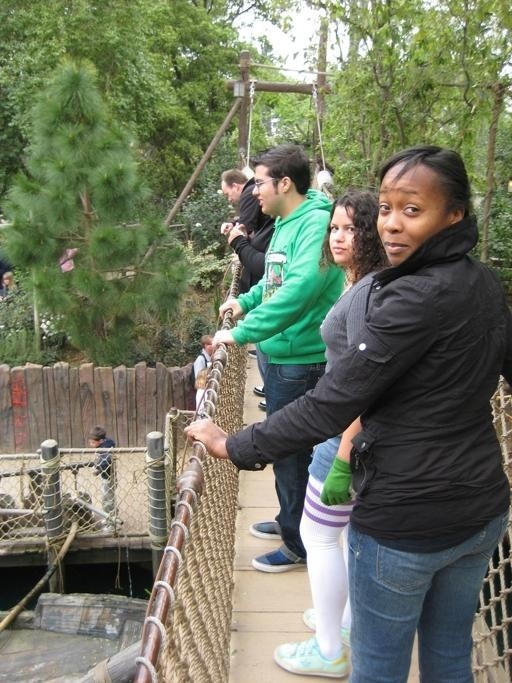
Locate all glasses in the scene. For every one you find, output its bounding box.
[252,175,279,188]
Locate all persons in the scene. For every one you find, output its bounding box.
[275,187,394,676]
[185,148,509,682]
[221,223,282,410]
[212,145,346,573]
[193,335,212,411]
[90,427,117,521]
[3,272,16,300]
[220,168,276,358]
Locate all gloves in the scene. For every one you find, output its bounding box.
[320,456,355,507]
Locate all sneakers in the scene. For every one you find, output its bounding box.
[248,543,307,574]
[246,516,285,540]
[257,399,267,411]
[272,634,351,680]
[300,605,355,646]
[247,349,257,359]
[253,386,266,397]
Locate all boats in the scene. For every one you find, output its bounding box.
[0,591,148,681]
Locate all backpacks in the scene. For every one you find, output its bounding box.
[189,353,209,393]
